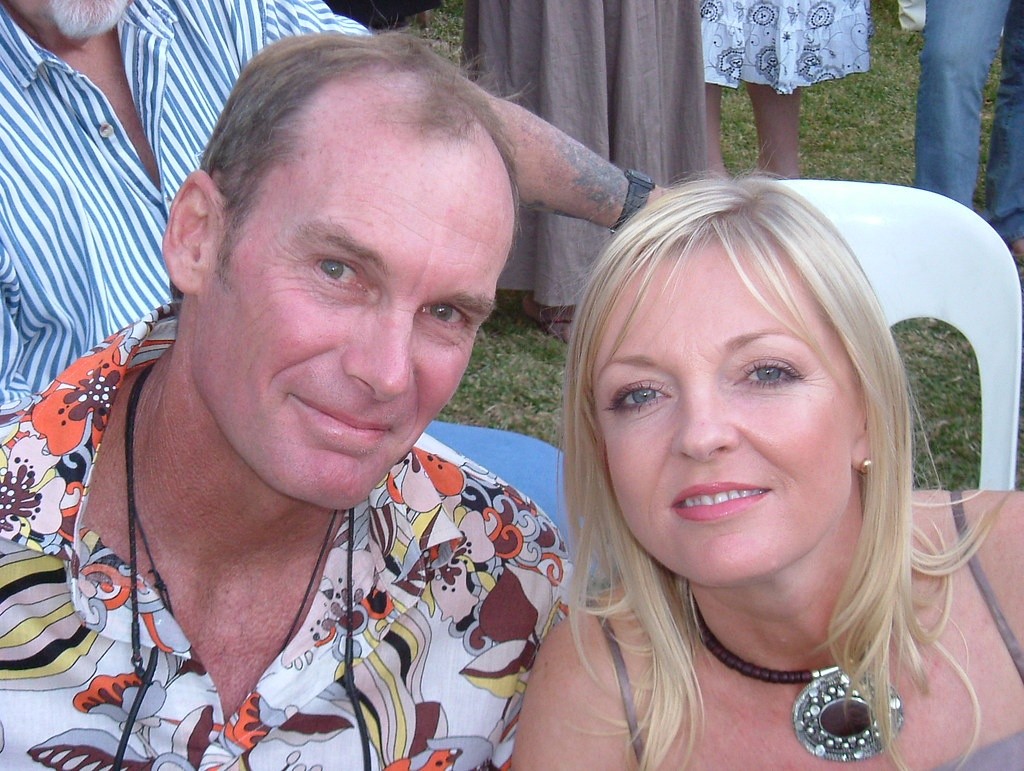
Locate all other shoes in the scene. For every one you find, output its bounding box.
[522,291,577,342]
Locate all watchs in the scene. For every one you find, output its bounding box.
[610,167,656,234]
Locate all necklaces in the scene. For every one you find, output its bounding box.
[137,508,340,655]
[111,360,371,771]
[677,576,904,764]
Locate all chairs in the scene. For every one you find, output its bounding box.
[776,178,1023,491]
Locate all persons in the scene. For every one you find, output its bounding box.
[504,182,1023,771]
[1,38,579,771]
[0,0,1024,414]
